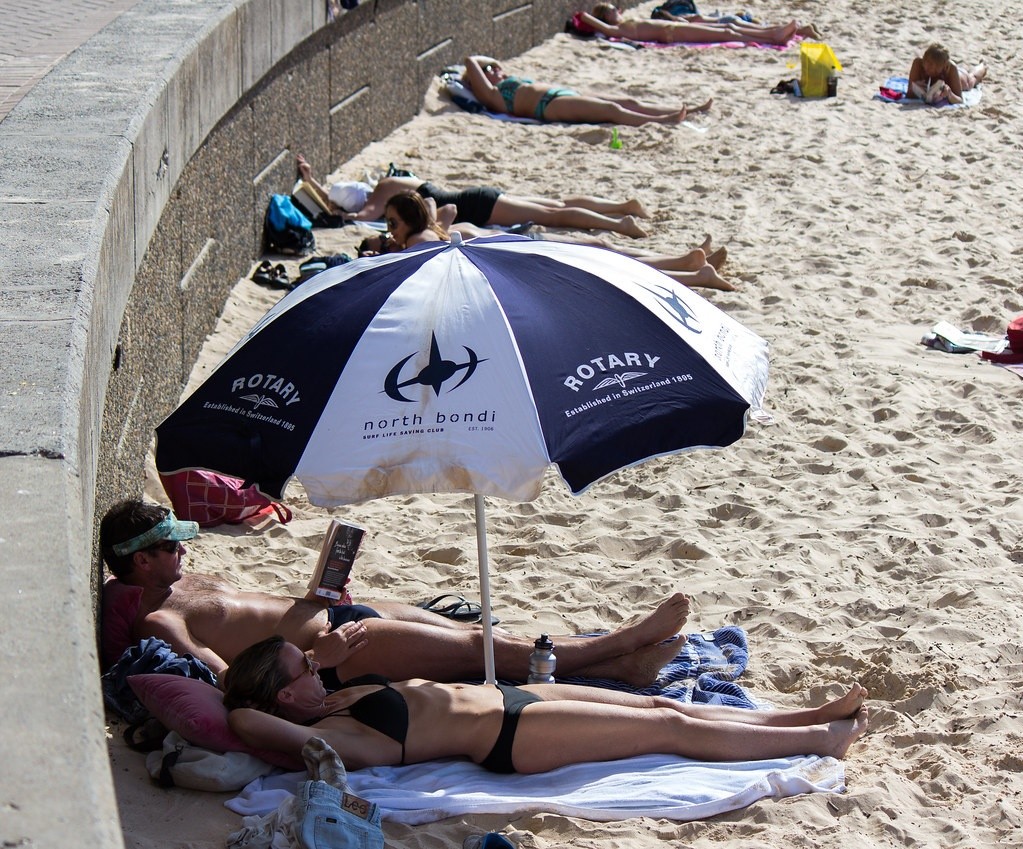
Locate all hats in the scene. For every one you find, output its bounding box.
[113,504,200,555]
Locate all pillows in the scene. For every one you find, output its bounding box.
[446,82,486,110]
[124,673,307,772]
[101,574,145,670]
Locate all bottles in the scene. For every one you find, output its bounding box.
[527,632,557,685]
[828,66,838,97]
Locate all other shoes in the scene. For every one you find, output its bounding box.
[462,829,516,849]
[272,263,289,285]
[253,259,272,283]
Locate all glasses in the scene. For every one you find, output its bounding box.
[291,649,312,683]
[383,217,398,229]
[612,6,618,14]
[482,64,499,73]
[154,540,180,553]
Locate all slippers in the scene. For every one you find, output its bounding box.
[415,593,501,626]
[484,220,534,235]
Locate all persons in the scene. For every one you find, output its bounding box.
[98,500,690,688]
[906,43,988,105]
[581,2,822,47]
[298,155,736,292]
[461,56,713,127]
[222,634,869,773]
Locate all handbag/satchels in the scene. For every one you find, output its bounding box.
[770,77,838,97]
[651,0,697,19]
[145,728,273,792]
[157,466,293,527]
[263,192,316,256]
[798,41,843,99]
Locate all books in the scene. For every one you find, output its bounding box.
[912,80,946,105]
[306,519,366,600]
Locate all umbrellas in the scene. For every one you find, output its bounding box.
[152,232,771,684]
